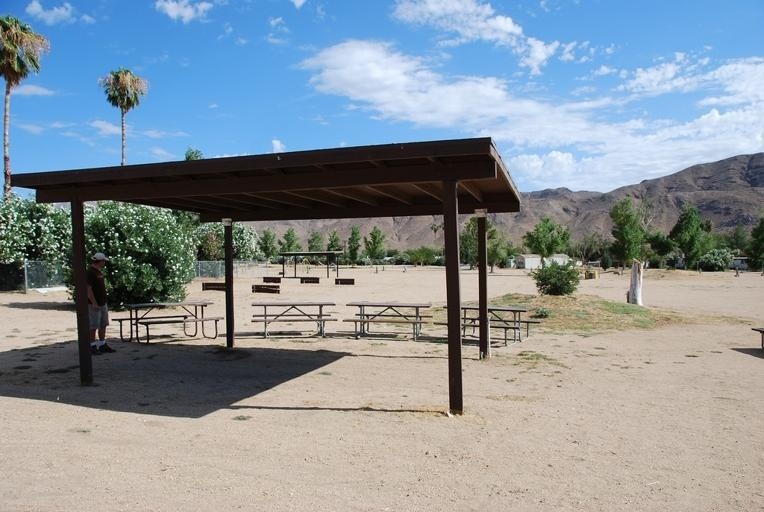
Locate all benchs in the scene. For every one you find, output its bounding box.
[251,319,338,338]
[111,315,198,342]
[355,314,434,332]
[137,316,224,344]
[463,317,540,337]
[253,313,331,334]
[343,319,428,341]
[434,319,521,346]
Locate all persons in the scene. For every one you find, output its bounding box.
[85,251,118,355]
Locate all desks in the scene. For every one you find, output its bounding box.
[123,300,214,344]
[251,301,335,338]
[345,302,431,339]
[443,302,527,346]
